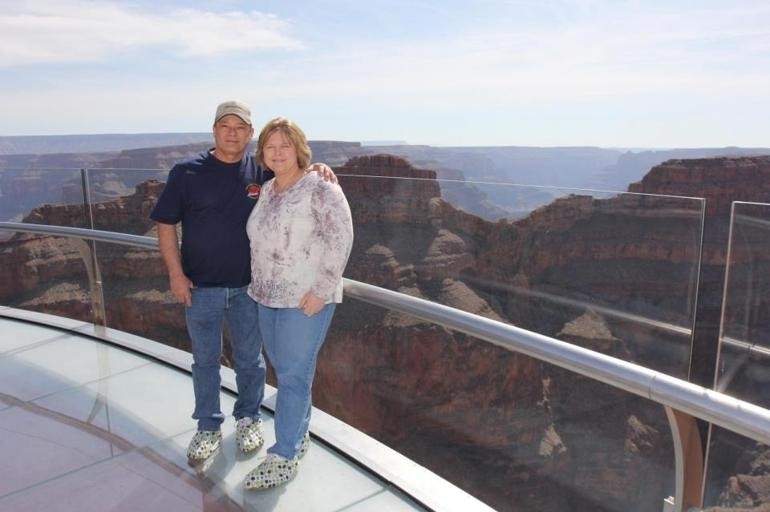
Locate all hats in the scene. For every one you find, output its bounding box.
[214,101,253,127]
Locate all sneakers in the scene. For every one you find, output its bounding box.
[267,431,311,462]
[186,430,223,461]
[234,416,265,454]
[243,454,298,492]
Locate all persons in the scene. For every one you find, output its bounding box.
[242,115,355,493]
[148,99,341,466]
